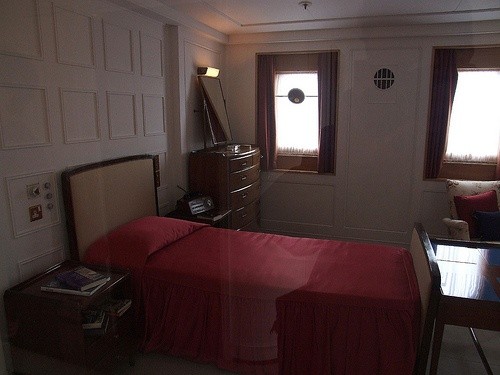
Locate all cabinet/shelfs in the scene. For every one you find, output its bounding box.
[188,143,263,232]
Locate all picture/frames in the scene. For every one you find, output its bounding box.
[16,247,66,283]
[5,170,58,238]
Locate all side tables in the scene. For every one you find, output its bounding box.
[173,205,234,228]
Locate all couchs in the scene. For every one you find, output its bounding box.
[443,178,500,244]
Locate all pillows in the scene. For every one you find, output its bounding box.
[453,190,500,241]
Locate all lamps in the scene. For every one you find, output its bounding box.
[197,66,220,77]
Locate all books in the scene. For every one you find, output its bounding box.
[40,265,111,296]
[81,297,134,332]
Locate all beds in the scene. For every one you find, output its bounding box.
[60,152,443,375]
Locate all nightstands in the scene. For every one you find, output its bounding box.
[4,259,145,375]
